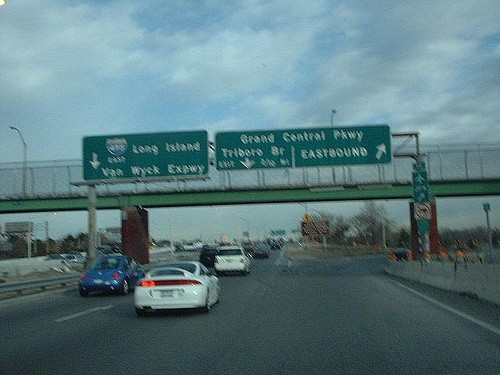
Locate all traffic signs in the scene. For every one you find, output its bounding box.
[214,124,393,171]
[81,129,210,180]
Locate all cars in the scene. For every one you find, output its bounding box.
[253,243,269,257]
[133,259,221,316]
[79,254,146,297]
[268,236,282,249]
[199,244,217,266]
[181,241,196,252]
[242,242,254,252]
[213,246,251,274]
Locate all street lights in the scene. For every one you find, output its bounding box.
[8,124,30,196]
